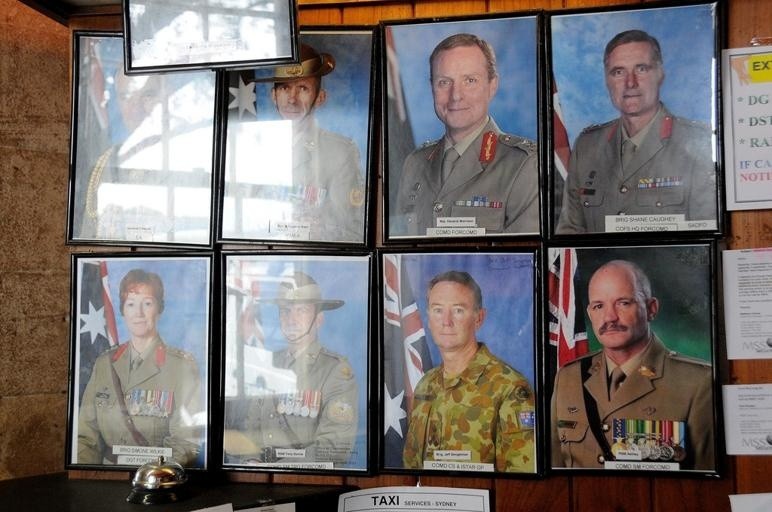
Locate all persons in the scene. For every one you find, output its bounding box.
[232,46,365,244]
[556,29,716,233]
[402,270,535,473]
[225,271,358,470]
[74,61,209,242]
[545,259,720,472]
[78,268,204,467]
[241,5,275,58]
[391,33,540,235]
[154,10,206,46]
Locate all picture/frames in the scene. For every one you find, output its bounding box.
[121,0,301,75]
[64,30,222,251]
[379,9,546,245]
[64,250,216,473]
[541,237,726,481]
[543,0,726,245]
[214,24,379,248]
[219,249,374,477]
[374,245,544,480]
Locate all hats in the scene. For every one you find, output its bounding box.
[253,45,335,83]
[256,272,345,311]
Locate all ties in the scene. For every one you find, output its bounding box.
[283,356,295,370]
[441,147,459,186]
[622,140,635,171]
[609,367,627,398]
[129,357,142,374]
